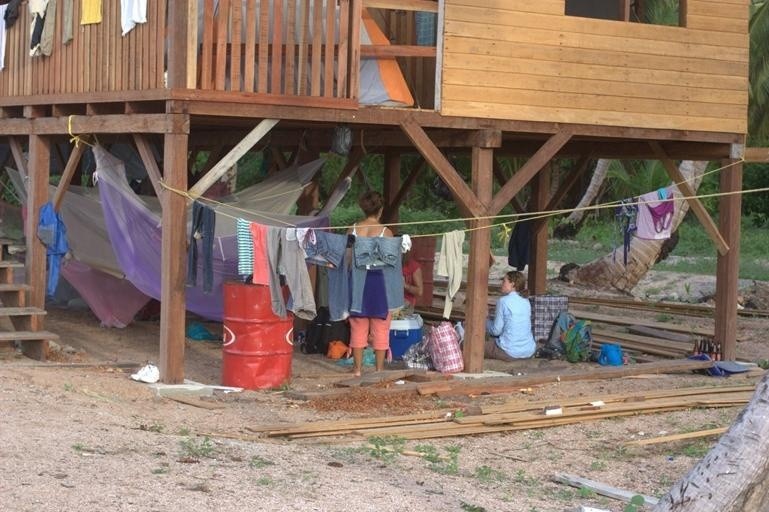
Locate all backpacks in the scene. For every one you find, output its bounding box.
[548,312,577,352]
[560,320,593,363]
[300,307,349,356]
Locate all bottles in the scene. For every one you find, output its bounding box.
[693,337,722,361]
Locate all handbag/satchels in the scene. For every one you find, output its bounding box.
[421,321,465,374]
[528,293,569,342]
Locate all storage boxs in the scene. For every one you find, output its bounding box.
[389,314,423,360]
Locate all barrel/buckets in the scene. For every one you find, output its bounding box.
[222,280,294,390]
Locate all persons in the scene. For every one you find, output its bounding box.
[482,272,536,360]
[393,233,424,312]
[342,191,401,378]
